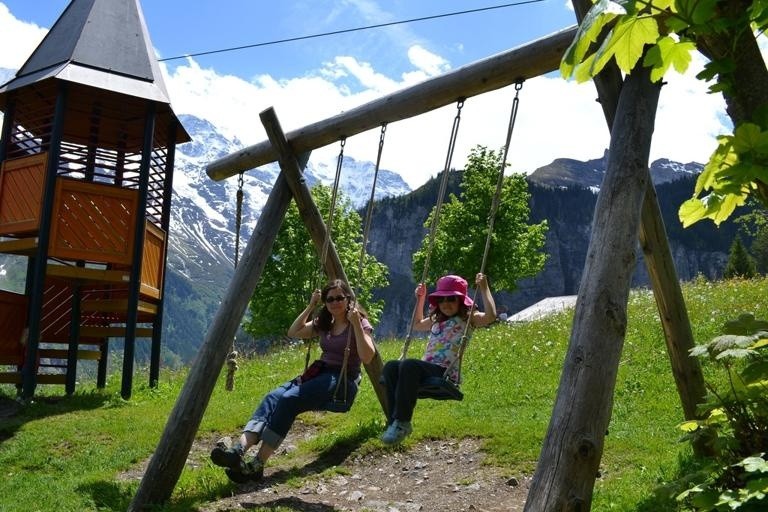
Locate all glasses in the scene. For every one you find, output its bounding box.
[433,295,458,305]
[325,295,349,303]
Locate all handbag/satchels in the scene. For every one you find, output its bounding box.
[301,359,326,384]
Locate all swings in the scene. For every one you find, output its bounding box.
[390,78,523,399]
[304,124,385,413]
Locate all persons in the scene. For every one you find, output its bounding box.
[209,277,376,485]
[378,272,498,446]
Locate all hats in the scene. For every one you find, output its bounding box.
[428,273,481,311]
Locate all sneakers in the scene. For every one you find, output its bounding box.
[224,457,263,482]
[210,442,244,469]
[383,420,413,445]
